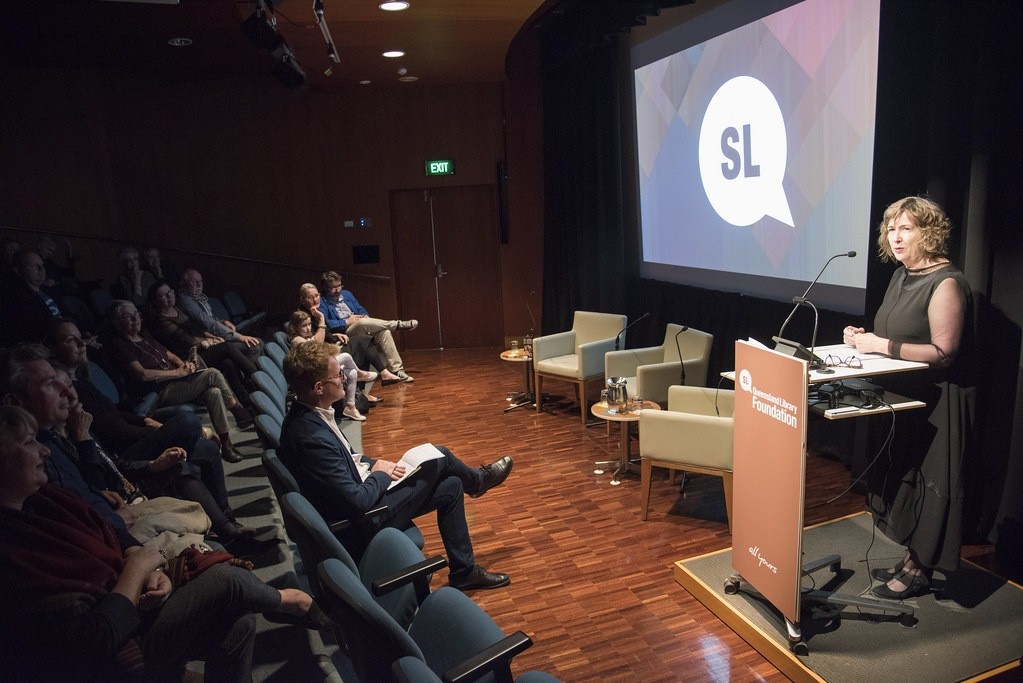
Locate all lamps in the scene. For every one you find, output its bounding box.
[239,0,306,90]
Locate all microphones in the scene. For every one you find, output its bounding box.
[675,326,688,384]
[772,251,856,370]
[614,312,651,350]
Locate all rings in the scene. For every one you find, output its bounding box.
[158,549,168,558]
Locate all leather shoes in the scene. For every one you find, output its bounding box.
[469,455,513,499]
[450,564,510,590]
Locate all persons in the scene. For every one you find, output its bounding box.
[841,196,980,600]
[0,225,340,683]
[286,311,379,421]
[316,267,420,383]
[292,283,409,404]
[278,340,516,590]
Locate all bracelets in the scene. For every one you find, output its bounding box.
[888,338,902,360]
[318,325,328,328]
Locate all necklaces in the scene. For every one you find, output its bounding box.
[128,335,170,370]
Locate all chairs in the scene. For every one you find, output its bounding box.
[532,310,627,425]
[0,265,541,683]
[637,385,735,534]
[604,323,714,435]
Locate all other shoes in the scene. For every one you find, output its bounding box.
[263,587,338,632]
[358,372,378,382]
[342,405,366,421]
[229,517,256,536]
[367,397,385,403]
[221,443,243,463]
[381,377,408,387]
[871,562,905,582]
[217,531,279,555]
[872,573,930,599]
[236,409,254,431]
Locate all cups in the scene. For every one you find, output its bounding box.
[511,341,519,353]
[632,396,643,414]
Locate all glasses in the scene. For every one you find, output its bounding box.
[312,368,345,390]
[159,289,174,301]
[824,354,863,369]
[305,293,321,300]
[329,284,344,290]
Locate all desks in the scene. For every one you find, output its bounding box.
[590,400,661,485]
[500,348,557,416]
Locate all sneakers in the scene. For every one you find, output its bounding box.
[397,320,418,331]
[395,370,414,383]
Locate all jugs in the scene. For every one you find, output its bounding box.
[600,377,628,413]
